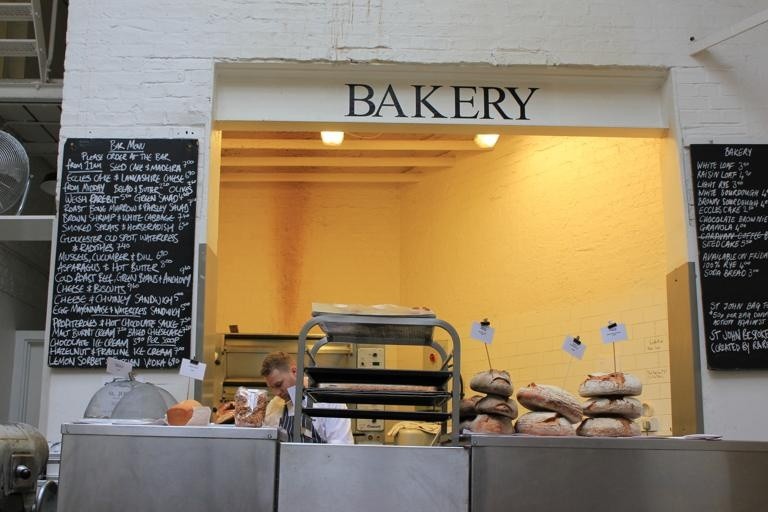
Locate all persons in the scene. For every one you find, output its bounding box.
[259,349,355,445]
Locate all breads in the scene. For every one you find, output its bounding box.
[581,396,642,419]
[168,400,202,425]
[459,397,478,418]
[578,371,642,397]
[578,415,642,437]
[470,414,516,435]
[213,400,236,424]
[470,370,514,397]
[474,393,519,420]
[513,412,576,436]
[518,383,583,424]
[459,419,473,434]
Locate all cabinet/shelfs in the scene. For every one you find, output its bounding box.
[294,314,459,447]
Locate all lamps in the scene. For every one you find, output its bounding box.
[321,131,345,145]
[40,173,56,196]
[473,133,499,148]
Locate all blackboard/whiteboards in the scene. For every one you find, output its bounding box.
[48,138,200,370]
[690,144,768,371]
